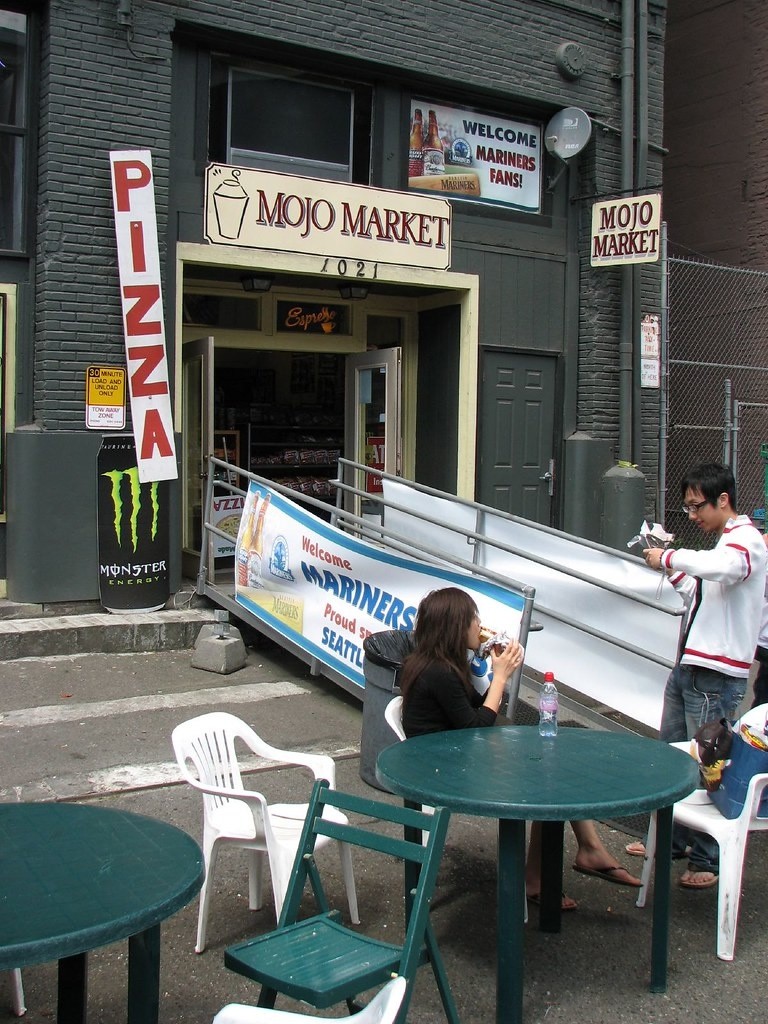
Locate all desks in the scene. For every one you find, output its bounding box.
[0,802,208,1024]
[374,724,700,1023]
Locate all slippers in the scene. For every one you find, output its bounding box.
[626,840,693,859]
[526,889,577,910]
[572,862,643,887]
[680,862,718,888]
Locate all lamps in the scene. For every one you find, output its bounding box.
[239,272,275,293]
[336,282,370,301]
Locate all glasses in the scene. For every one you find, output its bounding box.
[681,500,710,514]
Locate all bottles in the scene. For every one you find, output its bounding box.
[407,108,424,178]
[422,109,445,176]
[539,671,558,737]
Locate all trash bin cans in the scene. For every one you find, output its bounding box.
[358,630,415,794]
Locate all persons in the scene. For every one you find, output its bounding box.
[624,462,766,889]
[398,585,645,909]
[751,534,768,710]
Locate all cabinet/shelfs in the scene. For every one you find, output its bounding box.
[212,421,345,518]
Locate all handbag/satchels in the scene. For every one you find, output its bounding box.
[707,721,768,820]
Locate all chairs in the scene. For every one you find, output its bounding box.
[225,777,452,1024]
[385,696,532,924]
[211,975,405,1024]
[172,711,361,953]
[637,703,768,961]
